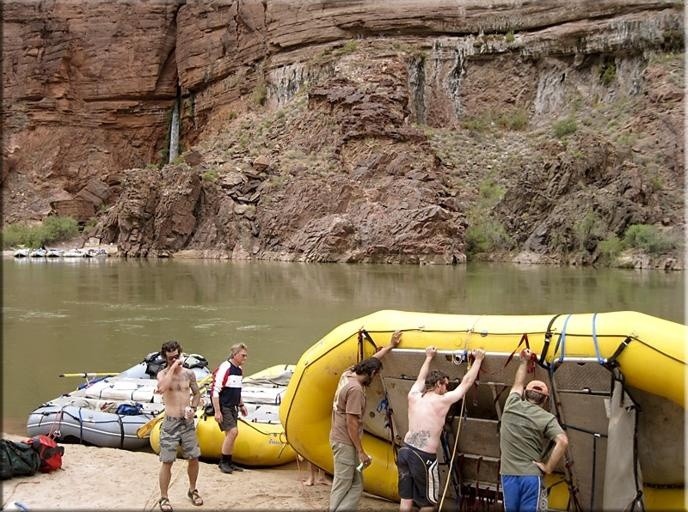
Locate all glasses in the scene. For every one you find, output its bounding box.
[168,355,178,360]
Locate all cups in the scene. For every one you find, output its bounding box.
[184,407,191,419]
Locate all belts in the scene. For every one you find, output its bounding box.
[168,417,184,421]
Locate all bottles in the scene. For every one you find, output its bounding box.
[356,456,373,472]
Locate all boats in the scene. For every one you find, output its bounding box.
[26,351,212,452]
[278,309,686,512]
[148,363,297,469]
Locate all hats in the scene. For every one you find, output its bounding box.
[525,380,548,395]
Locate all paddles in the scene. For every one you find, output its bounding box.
[136,383,210,440]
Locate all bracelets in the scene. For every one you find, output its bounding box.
[189,405,197,412]
[239,404,245,408]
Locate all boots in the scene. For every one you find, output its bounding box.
[230,455,241,471]
[219,454,232,474]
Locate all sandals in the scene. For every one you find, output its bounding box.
[159,497,173,512]
[188,488,203,505]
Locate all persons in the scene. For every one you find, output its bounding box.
[304,460,330,486]
[155,339,203,512]
[397,345,486,512]
[498,349,568,512]
[210,343,248,474]
[329,328,403,512]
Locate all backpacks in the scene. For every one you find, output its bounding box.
[0,440,40,480]
[21,434,64,473]
